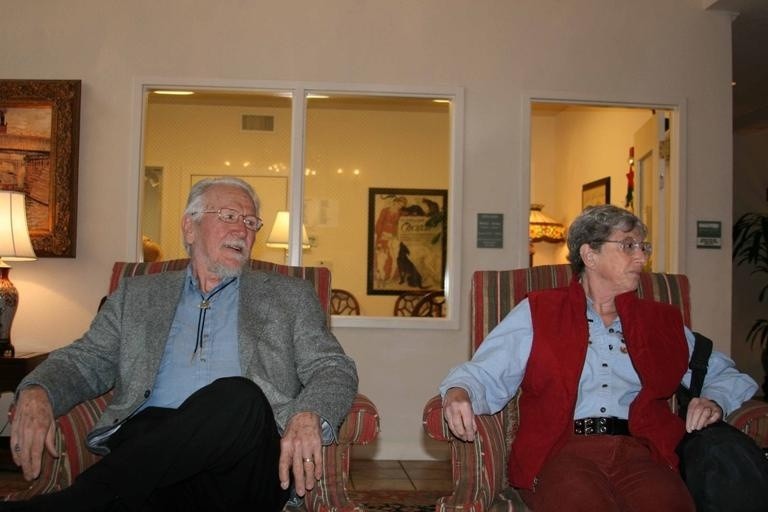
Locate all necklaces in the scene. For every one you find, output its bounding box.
[590,299,619,316]
[189,275,238,361]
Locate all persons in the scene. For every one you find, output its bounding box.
[439,204,761,511]
[1,174,359,512]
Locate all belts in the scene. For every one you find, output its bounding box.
[572,417,630,436]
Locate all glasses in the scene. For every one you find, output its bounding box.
[193,206,265,232]
[590,237,653,257]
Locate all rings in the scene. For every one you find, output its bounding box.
[303,457,313,463]
[14,446,20,451]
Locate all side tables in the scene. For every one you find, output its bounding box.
[0,353,51,473]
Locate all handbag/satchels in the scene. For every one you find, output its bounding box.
[675,401,768,512]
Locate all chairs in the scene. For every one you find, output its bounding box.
[329,289,360,315]
[412,290,448,317]
[4,258,380,512]
[393,292,427,316]
[422,264,768,512]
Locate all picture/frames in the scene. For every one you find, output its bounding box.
[188,173,290,265]
[581,175,611,210]
[367,187,448,296]
[0,77,82,260]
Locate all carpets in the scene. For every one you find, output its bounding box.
[347,489,451,512]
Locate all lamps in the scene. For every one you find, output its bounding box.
[264,212,310,265]
[528,203,568,267]
[0,190,37,355]
